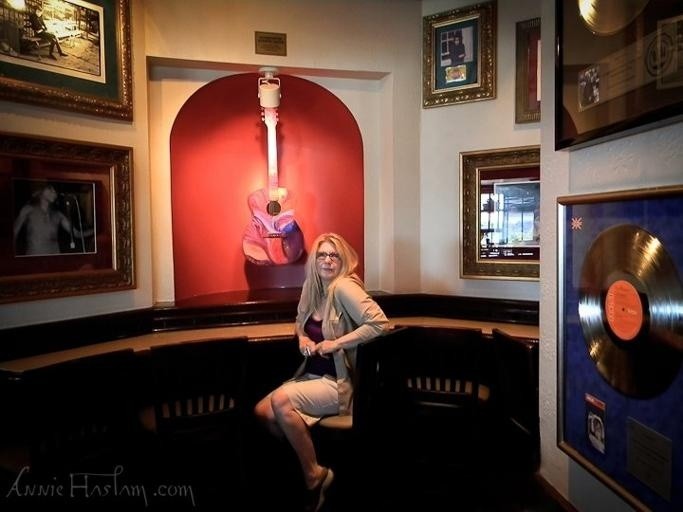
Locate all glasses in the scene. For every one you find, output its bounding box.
[316,251,340,263]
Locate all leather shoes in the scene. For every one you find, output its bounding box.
[303,467,334,512]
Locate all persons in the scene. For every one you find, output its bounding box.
[12,181,94,255]
[255,229,392,512]
[449,34,466,65]
[29,5,68,60]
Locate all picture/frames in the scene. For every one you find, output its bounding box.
[459,145,540,282]
[420,0,497,109]
[0,130,137,305]
[555,181,682,511]
[554,0,683,152]
[515,16,542,122]
[0,0,133,122]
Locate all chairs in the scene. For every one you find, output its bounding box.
[0,323,541,511]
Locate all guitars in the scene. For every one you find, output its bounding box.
[243,107,304,267]
